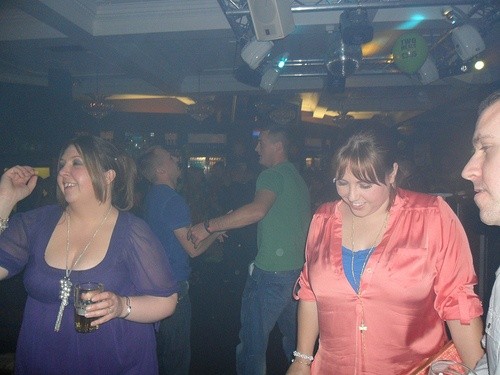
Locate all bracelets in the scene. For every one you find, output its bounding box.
[204,219,212,234]
[292,351,314,360]
[291,359,311,366]
[0,216,10,231]
[122,295,132,319]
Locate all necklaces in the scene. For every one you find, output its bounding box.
[351,208,389,330]
[54,204,111,333]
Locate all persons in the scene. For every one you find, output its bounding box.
[395,155,436,193]
[187,117,310,375]
[285,123,482,375]
[462,90,500,375]
[137,145,229,375]
[0,129,178,375]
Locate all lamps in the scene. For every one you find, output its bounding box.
[269,91,297,126]
[185,70,214,124]
[451,24,486,60]
[332,110,354,125]
[80,62,116,122]
[260,68,278,89]
[243,37,275,70]
[325,32,363,78]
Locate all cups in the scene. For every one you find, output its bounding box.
[74,282,104,333]
[429,360,477,375]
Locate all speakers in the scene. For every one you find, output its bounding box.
[247,0,295,41]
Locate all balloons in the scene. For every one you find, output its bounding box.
[393,31,429,75]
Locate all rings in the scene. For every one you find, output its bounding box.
[108,307,112,313]
